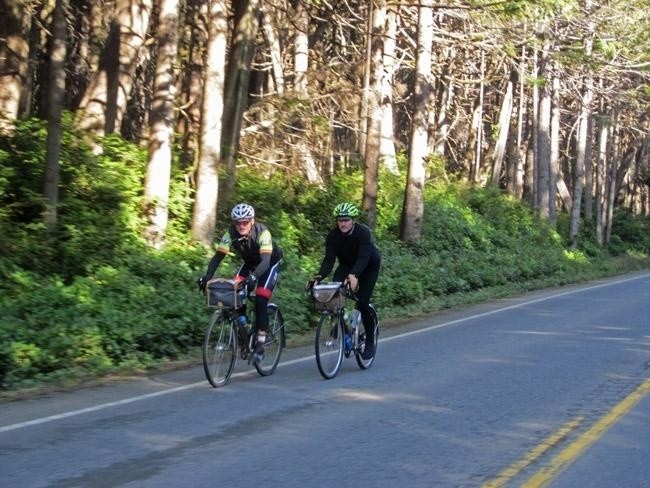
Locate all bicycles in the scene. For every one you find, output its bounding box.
[196,278,285,386]
[307,275,380,381]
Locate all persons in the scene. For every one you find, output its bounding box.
[304,201,382,360]
[197,202,284,361]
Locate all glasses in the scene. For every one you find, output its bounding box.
[235,221,250,227]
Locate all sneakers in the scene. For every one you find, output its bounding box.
[244,324,266,360]
[362,341,376,360]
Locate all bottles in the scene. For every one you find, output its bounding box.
[352,328,358,346]
[248,310,255,324]
[345,336,352,349]
[240,316,249,332]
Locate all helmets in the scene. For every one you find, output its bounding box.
[231,202,255,221]
[333,202,359,218]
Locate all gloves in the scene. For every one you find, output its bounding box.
[344,274,359,293]
[306,276,322,289]
[243,274,256,295]
[198,276,208,293]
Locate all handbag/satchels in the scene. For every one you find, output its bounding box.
[311,284,345,311]
[205,278,244,309]
